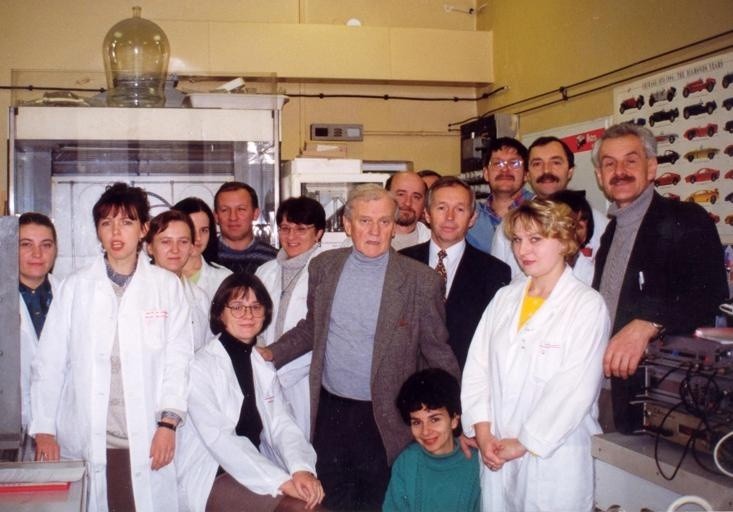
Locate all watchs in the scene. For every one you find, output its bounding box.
[651,321,665,335]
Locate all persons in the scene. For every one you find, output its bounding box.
[18,136,609,512]
[591,123,729,436]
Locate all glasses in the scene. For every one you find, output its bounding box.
[218,301,266,320]
[276,223,315,236]
[486,158,526,172]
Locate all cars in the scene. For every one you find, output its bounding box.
[616,71,732,233]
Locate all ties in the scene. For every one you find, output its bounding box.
[433,249,449,302]
